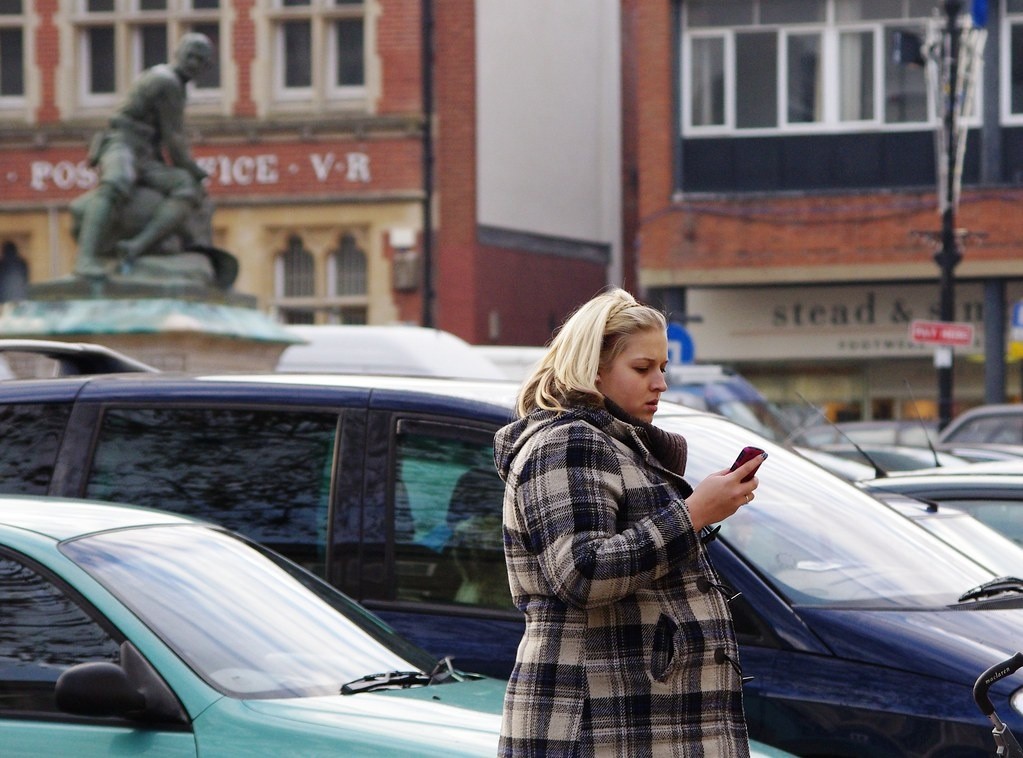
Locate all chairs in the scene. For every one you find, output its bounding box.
[447,469,503,603]
[390,477,438,602]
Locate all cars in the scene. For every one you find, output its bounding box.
[673,359,1023,587]
[0,489,798,758]
[0,368,1023,758]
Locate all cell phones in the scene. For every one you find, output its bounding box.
[726,446,765,483]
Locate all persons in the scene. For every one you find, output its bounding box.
[448,468,514,608]
[73,34,213,275]
[494,287,767,758]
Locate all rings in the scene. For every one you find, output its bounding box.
[746,495,749,504]
